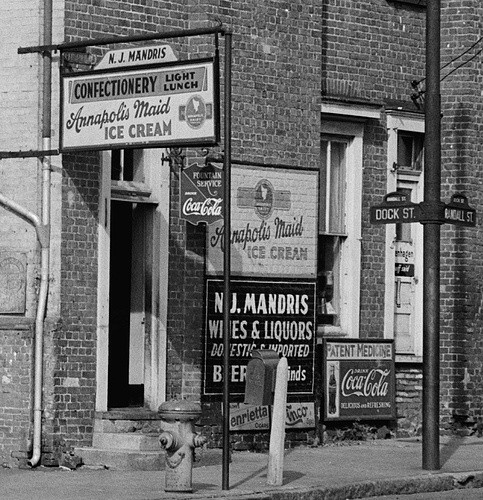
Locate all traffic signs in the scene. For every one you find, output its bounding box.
[440,204,479,228]
[369,203,421,225]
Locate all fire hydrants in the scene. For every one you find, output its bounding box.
[156,399,208,492]
